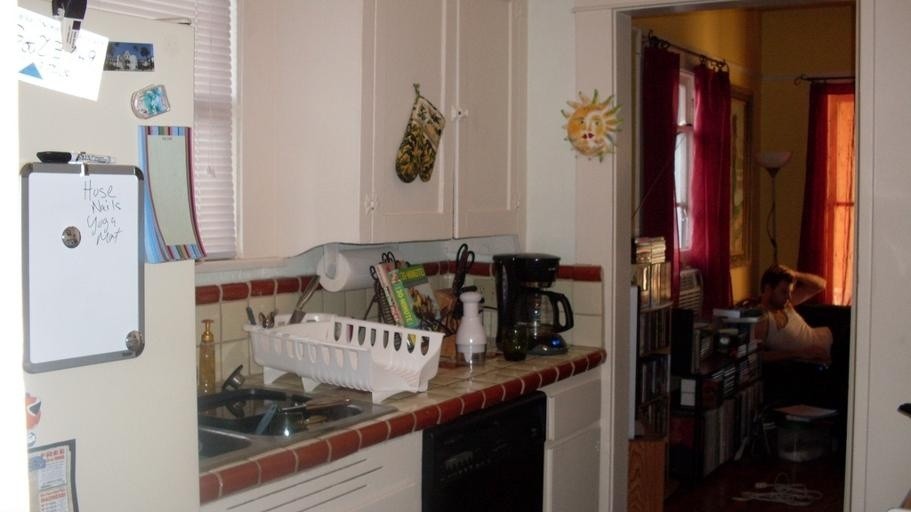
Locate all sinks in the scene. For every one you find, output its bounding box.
[195,384,398,438]
[197,424,253,476]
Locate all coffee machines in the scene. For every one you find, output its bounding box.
[494,252,574,356]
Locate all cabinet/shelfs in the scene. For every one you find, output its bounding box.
[630,241,760,492]
[234,0,529,263]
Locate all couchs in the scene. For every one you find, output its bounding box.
[744,301,848,429]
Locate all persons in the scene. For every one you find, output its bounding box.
[752,263,851,363]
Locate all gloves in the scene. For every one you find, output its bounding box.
[395,95,446,183]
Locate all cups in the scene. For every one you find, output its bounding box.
[503,327,528,360]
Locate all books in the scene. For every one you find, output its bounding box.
[631,234,674,442]
[773,404,839,422]
[700,308,765,478]
[375,261,451,350]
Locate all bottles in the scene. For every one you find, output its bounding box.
[200,319,216,391]
[455,291,488,366]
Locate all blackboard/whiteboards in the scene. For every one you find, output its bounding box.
[21,163,144,374]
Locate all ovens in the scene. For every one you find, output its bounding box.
[421,391,547,511]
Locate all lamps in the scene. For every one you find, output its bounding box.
[750,127,793,264]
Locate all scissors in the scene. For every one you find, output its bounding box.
[453,243,475,274]
[382,251,397,264]
